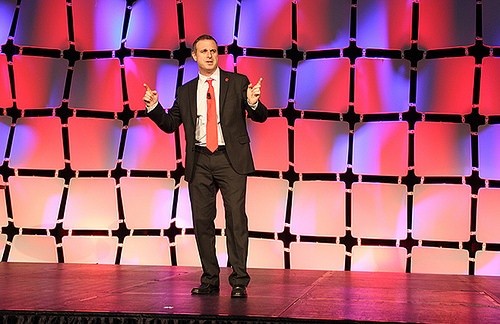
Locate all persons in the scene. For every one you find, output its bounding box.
[143,34,269,299]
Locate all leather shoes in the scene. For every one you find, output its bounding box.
[231,285,247,298]
[191,283,220,295]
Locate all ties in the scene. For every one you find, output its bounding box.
[205,79,218,153]
